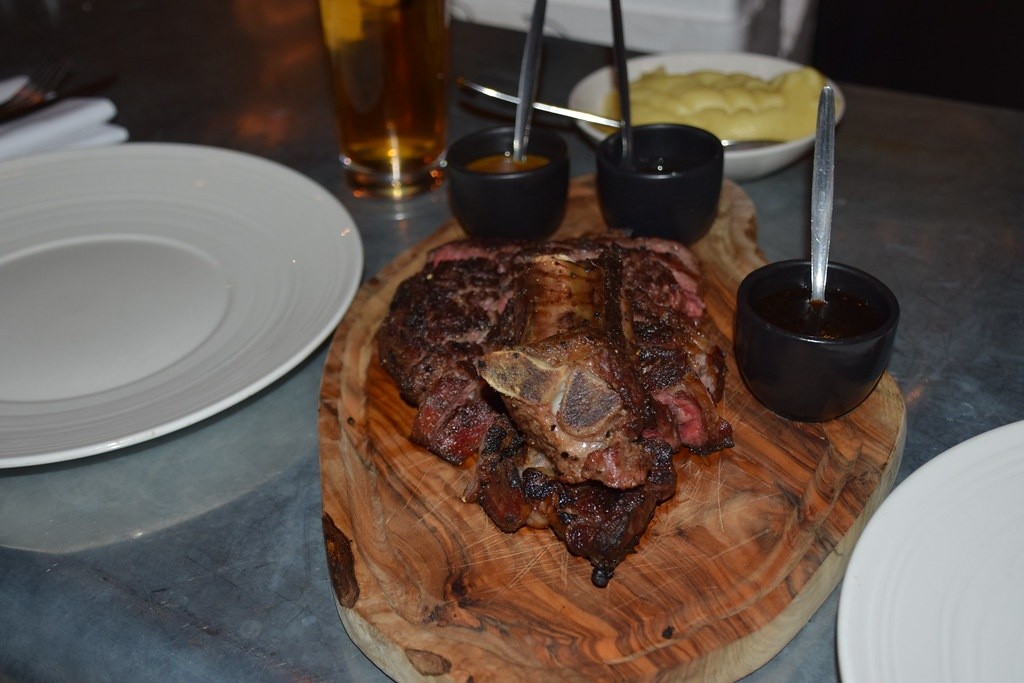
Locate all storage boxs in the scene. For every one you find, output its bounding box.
[446,0,817,63]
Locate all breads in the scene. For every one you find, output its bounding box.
[605,67,834,144]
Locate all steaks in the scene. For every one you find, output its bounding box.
[378,230,732,598]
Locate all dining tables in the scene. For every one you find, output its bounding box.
[0,0,1024,683]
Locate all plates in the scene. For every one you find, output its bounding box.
[567,51,845,181]
[0,142,364,473]
[318,171,907,683]
[836,419,1024,683]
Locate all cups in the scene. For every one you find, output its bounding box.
[732,258,901,423]
[443,125,572,245]
[319,0,450,203]
[596,122,724,247]
